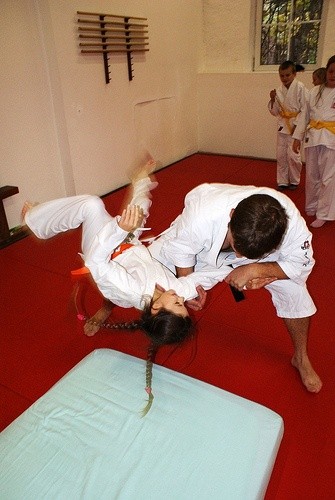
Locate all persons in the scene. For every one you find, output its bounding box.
[20,159,197,418]
[267,61,308,191]
[311,67,328,86]
[82,182,324,393]
[291,55,335,228]
